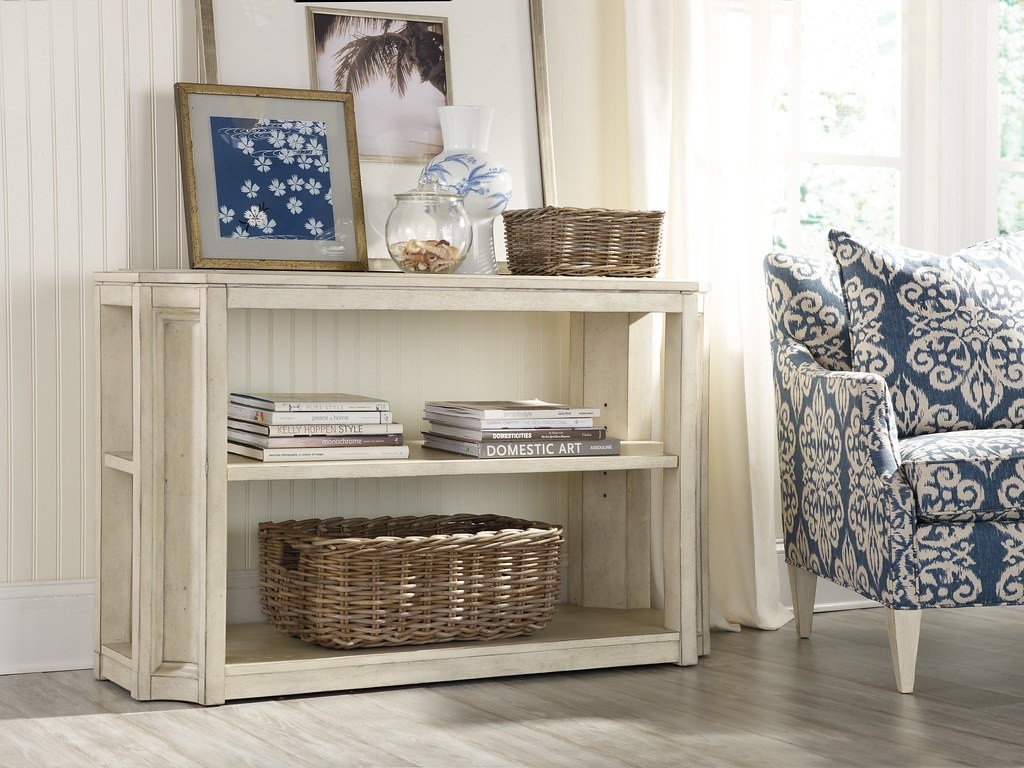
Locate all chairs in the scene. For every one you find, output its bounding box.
[766,251,1023,694]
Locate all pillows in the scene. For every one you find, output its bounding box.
[828,228,1024,438]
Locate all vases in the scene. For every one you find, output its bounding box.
[419,107,512,275]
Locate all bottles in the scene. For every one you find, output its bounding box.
[385,170,474,273]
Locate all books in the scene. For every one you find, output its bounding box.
[229,391,412,461]
[420,398,622,459]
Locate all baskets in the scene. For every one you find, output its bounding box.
[501,205,665,278]
[258,514,565,649]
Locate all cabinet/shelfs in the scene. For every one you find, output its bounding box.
[89,271,709,706]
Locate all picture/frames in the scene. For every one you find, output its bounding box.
[175,83,370,272]
[306,6,453,164]
